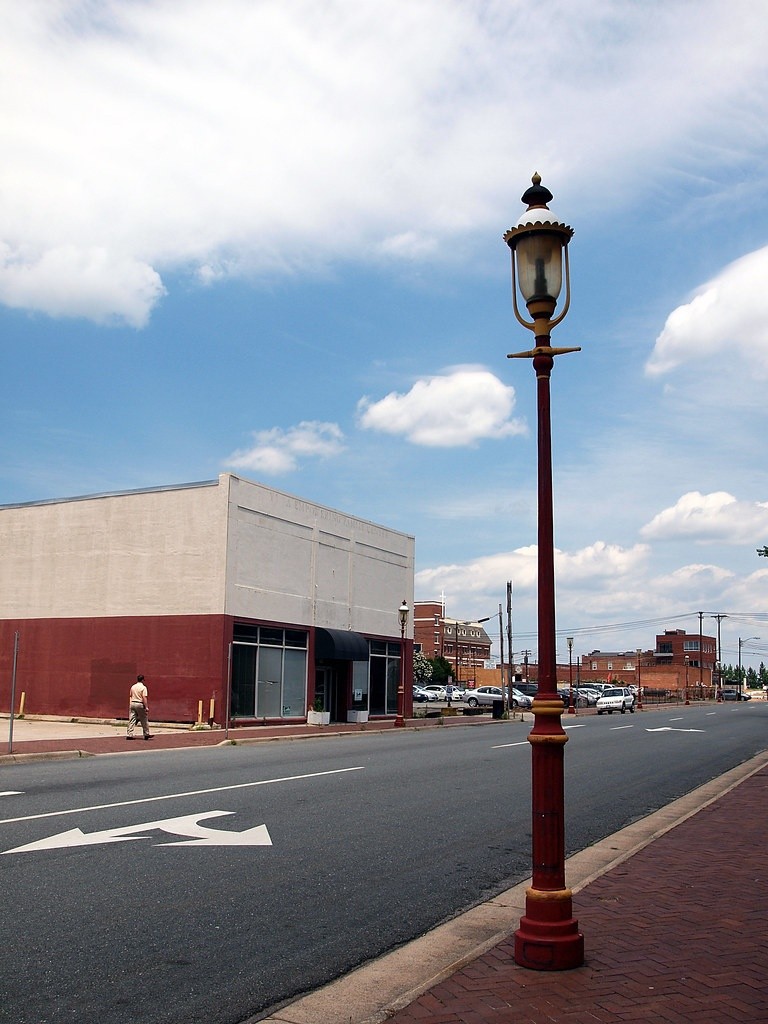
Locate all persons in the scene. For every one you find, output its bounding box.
[693,689,715,699]
[126,674,155,740]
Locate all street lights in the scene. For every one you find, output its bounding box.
[394,600,409,727]
[739,637,760,701]
[636,649,643,708]
[567,638,576,713]
[502,172,585,971]
[685,655,690,705]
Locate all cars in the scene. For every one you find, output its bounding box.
[721,689,751,701]
[462,681,538,708]
[413,685,474,703]
[557,681,644,715]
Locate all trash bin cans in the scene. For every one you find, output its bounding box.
[492,699,507,719]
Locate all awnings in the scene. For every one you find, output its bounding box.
[315,627,370,661]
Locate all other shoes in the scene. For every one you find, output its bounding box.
[144,734,154,740]
[126,737,136,740]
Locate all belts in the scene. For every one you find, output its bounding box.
[132,701,143,703]
